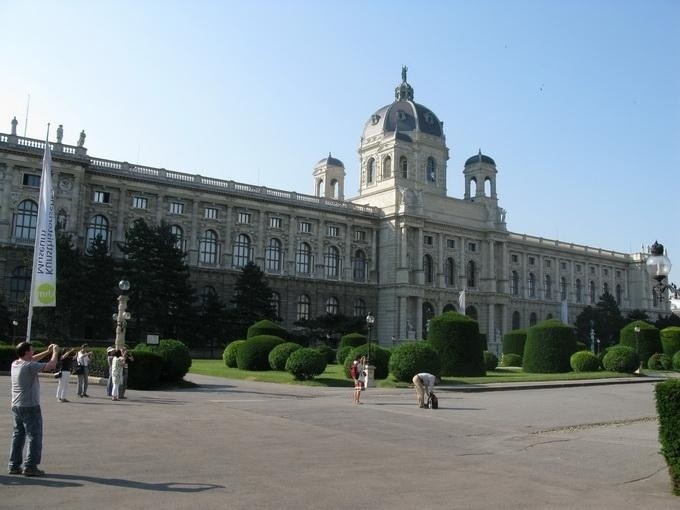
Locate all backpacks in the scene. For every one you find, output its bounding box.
[427,394,438,408]
[351,362,360,379]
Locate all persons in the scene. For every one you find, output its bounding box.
[7,342,59,477]
[76,343,95,398]
[56,346,78,403]
[413,373,442,408]
[105,345,135,401]
[352,354,366,406]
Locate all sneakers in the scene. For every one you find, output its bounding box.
[8,466,44,475]
[76,393,88,398]
[107,393,127,402]
[57,399,69,403]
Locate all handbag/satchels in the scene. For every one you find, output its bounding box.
[54,372,62,378]
[72,365,85,376]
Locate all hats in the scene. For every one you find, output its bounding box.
[107,347,116,353]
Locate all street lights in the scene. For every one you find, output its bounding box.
[112,279,131,369]
[365,310,378,366]
[11,318,19,347]
[634,323,643,378]
